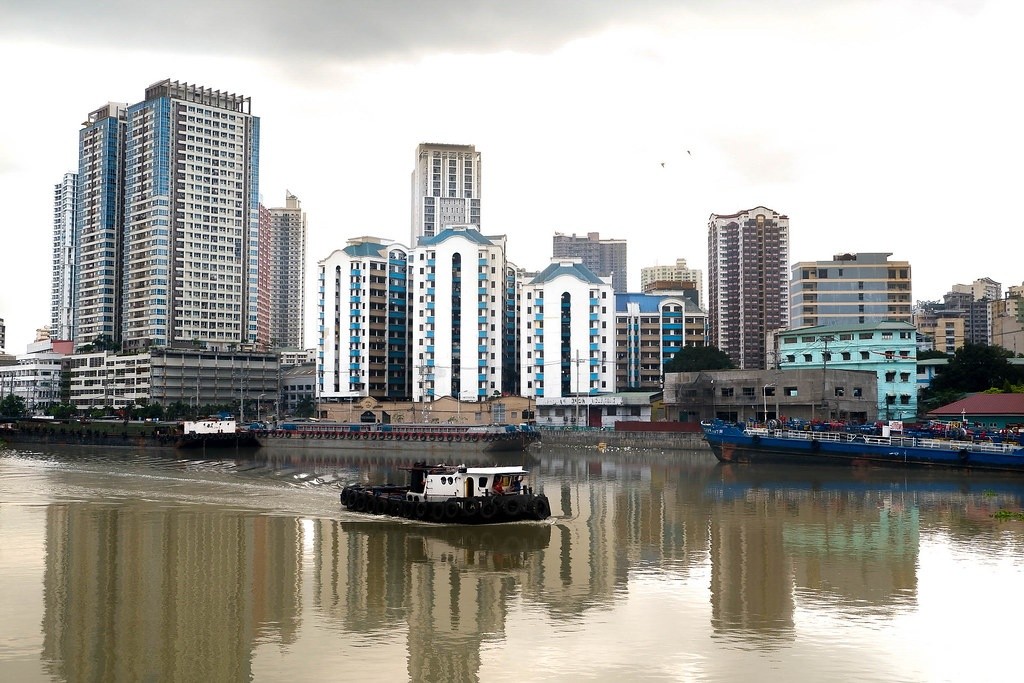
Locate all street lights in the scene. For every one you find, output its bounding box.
[763,384,770,423]
[458,389,469,423]
[258,393,266,421]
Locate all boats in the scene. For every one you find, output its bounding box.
[699,414,1024,472]
[337,458,553,527]
[247,396,541,451]
[0,419,260,449]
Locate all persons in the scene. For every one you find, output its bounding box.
[493,475,523,496]
